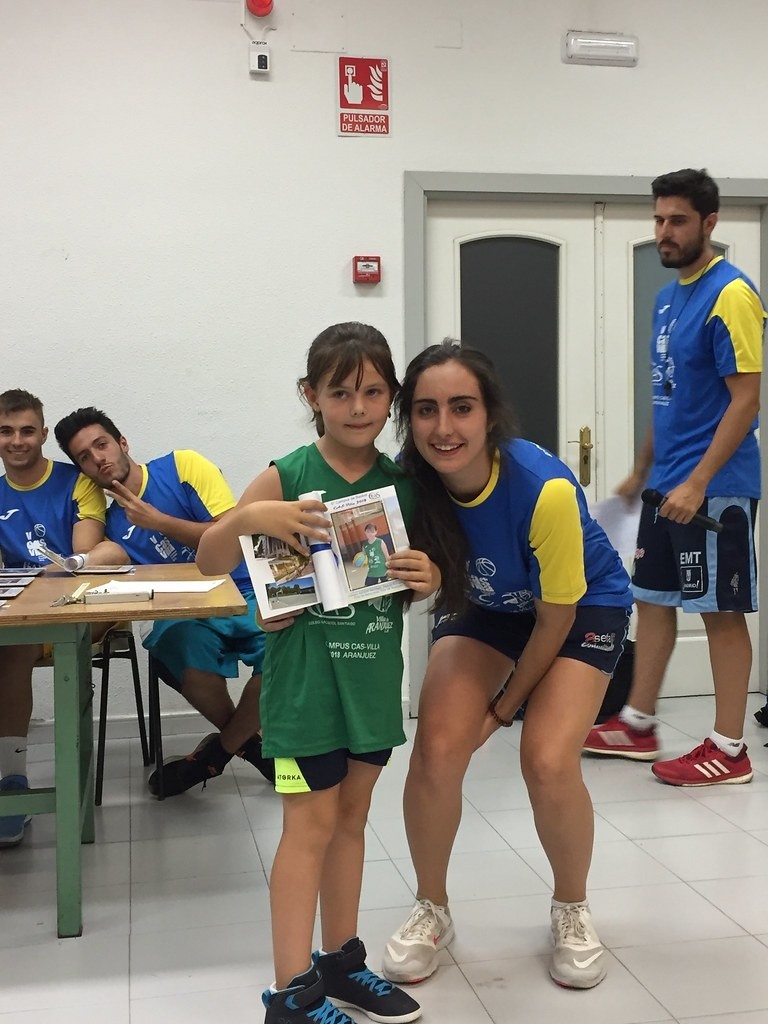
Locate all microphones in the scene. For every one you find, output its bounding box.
[641,489,723,534]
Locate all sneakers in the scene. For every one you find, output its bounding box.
[0,776,32,845]
[262,963,360,1024]
[148,732,222,797]
[382,899,454,982]
[234,733,276,783]
[313,936,424,1024]
[583,718,659,760]
[653,737,752,786]
[549,903,607,988]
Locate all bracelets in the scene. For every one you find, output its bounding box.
[254,608,268,633]
[490,700,513,727]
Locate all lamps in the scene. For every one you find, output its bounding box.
[561,31,639,67]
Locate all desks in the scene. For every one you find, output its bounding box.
[0,563,247,938]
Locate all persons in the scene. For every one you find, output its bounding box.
[256,336,634,991]
[195,321,442,1024]
[53,406,279,800]
[581,169,768,786]
[362,524,391,587]
[0,388,131,849]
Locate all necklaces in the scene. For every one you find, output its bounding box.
[662,256,714,397]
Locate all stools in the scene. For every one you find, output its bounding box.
[33,618,150,806]
